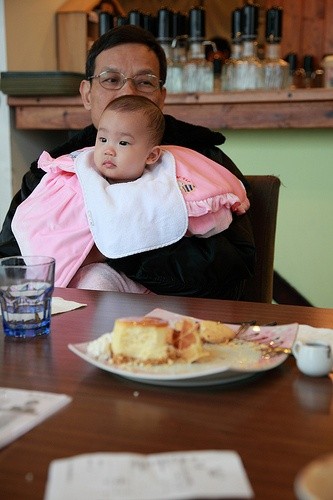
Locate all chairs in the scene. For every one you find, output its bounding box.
[240,175,281,304]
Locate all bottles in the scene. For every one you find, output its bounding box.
[98,5,316,93]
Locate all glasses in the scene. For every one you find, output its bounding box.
[88,70,163,93]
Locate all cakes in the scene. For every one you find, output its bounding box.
[111,317,203,367]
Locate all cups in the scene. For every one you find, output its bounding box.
[292,341,331,376]
[0,255,55,339]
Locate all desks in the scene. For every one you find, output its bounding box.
[0,286,333,500]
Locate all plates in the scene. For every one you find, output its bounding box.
[68,308,299,387]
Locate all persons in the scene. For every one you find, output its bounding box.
[0,23,257,303]
[11,95,251,288]
